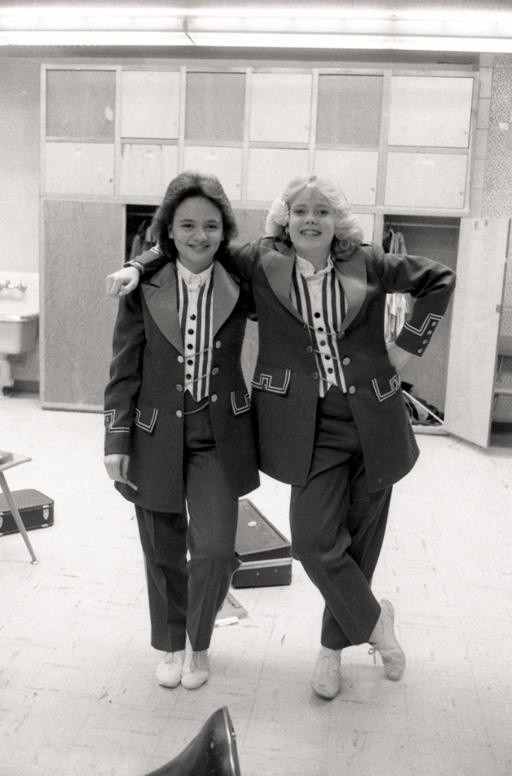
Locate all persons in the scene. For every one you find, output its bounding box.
[103,172,258,692]
[104,177,458,701]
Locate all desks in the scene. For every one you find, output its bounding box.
[0,447,43,568]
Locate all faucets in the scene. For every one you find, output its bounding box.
[0,280,26,291]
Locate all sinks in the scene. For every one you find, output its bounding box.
[0,301,39,355]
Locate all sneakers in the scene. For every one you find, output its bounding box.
[157,648,183,691]
[313,648,343,702]
[370,599,407,684]
[181,648,210,690]
